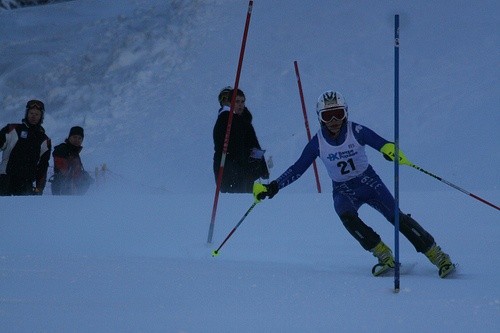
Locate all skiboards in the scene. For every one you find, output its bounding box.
[372,243,457,278]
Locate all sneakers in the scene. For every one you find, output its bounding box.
[369,241,392,264]
[426,243,451,272]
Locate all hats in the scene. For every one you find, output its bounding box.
[68,126,84,139]
[26,101,44,123]
[218,86,233,107]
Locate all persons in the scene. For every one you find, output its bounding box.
[212,85,270,193]
[253,90,456,279]
[51,126,95,196]
[0,100,52,196]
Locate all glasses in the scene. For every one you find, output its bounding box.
[27,100,43,110]
[219,92,231,100]
[319,106,347,123]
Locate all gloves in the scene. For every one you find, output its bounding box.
[258,180,279,200]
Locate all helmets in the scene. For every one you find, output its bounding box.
[316,90,348,121]
